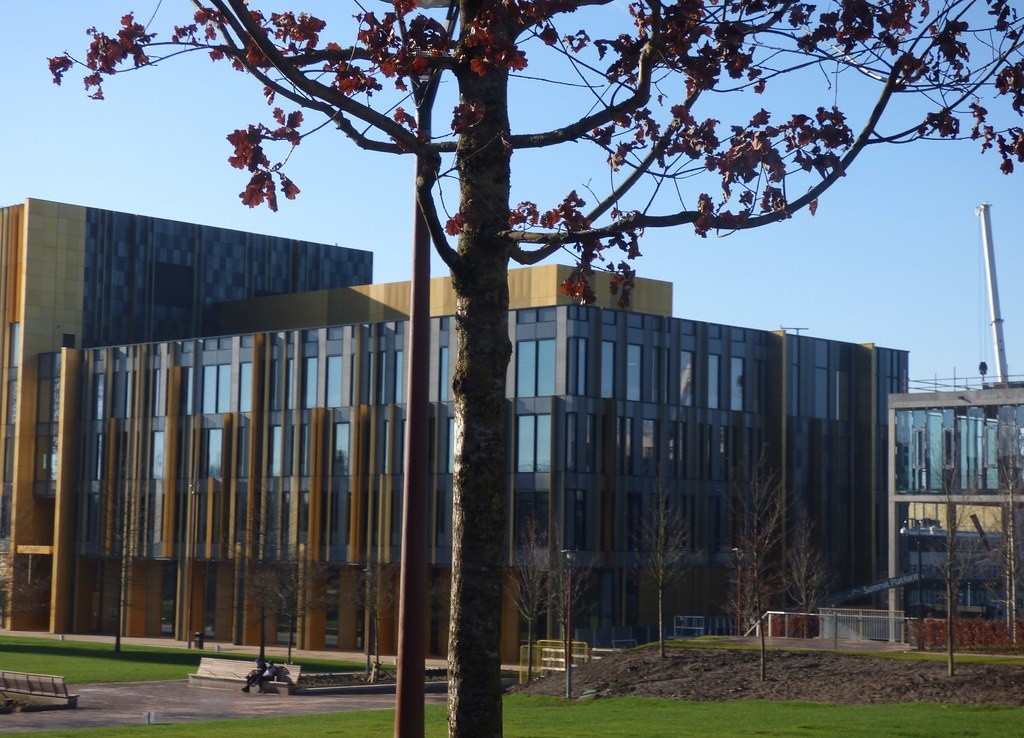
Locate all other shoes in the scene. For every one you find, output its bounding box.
[242,688,250,692]
[258,688,264,693]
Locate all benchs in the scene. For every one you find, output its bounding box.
[0,669,80,710]
[187,656,301,696]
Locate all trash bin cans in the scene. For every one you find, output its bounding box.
[193,632,204,649]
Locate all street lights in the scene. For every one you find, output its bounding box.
[733,548,743,638]
[900,516,938,651]
[361,565,378,672]
[559,547,580,700]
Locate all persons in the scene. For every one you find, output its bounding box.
[241,659,267,693]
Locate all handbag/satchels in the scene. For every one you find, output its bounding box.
[245,671,257,679]
[263,669,271,677]
